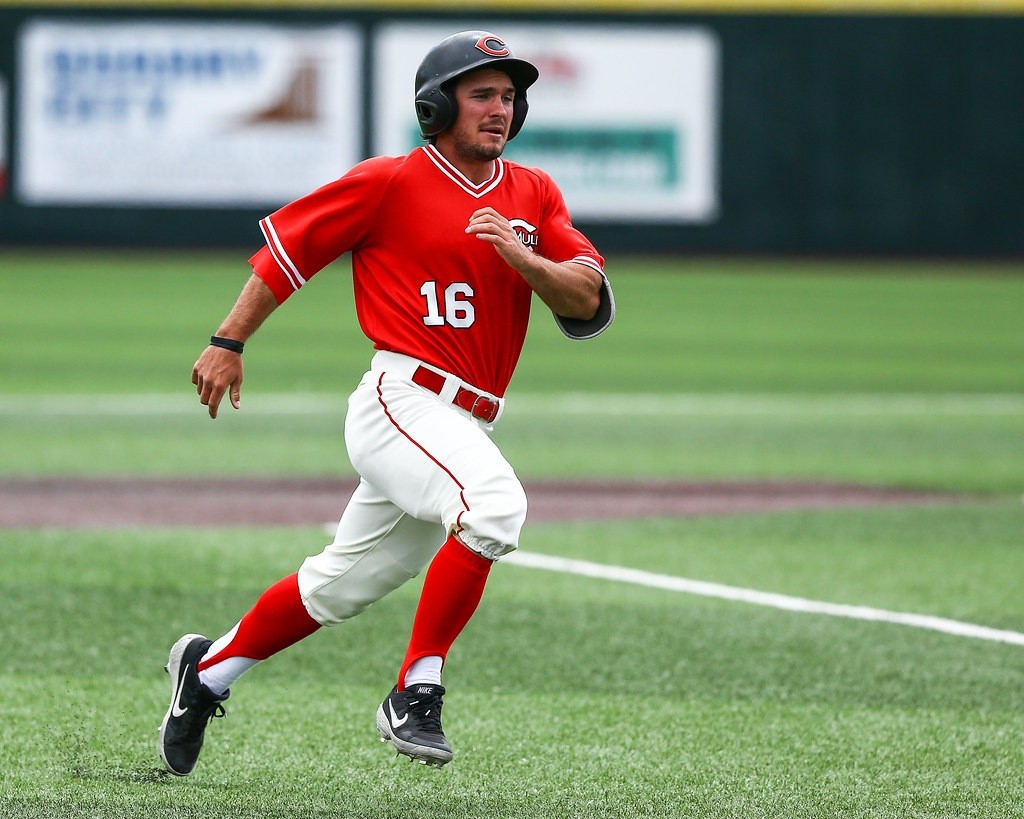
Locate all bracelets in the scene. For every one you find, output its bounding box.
[209,336,245,353]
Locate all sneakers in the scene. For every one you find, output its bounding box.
[158,631,231,776]
[376,679,454,772]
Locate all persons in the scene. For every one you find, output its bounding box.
[155,29,616,777]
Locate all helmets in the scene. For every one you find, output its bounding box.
[415,29,538,142]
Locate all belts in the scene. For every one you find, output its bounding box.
[411,371,502,426]
